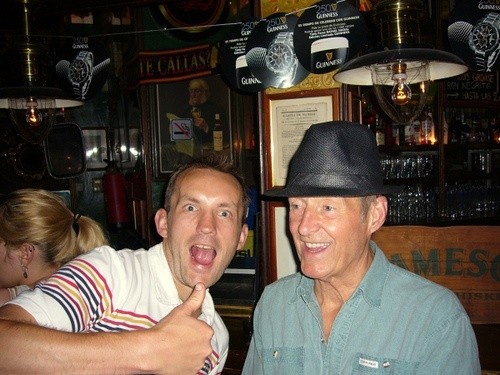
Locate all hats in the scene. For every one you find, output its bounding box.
[262,120,404,198]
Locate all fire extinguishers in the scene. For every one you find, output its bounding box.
[102,158,126,231]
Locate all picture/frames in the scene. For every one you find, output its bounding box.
[80,126,109,170]
[150,76,237,181]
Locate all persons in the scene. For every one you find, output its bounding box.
[240,121,481,375]
[0,153,250,374]
[0,188,112,308]
[170,79,215,164]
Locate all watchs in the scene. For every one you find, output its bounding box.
[67,51,94,100]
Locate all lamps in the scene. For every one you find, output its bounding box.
[0,0,84,139]
[332,0,468,127]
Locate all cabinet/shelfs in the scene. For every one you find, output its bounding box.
[355,0,500,226]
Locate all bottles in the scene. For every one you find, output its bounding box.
[212,113,224,152]
[362,90,438,153]
[443,109,500,145]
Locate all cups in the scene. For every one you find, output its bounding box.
[382,182,434,218]
[377,152,434,179]
[440,151,500,220]
[192,106,201,118]
